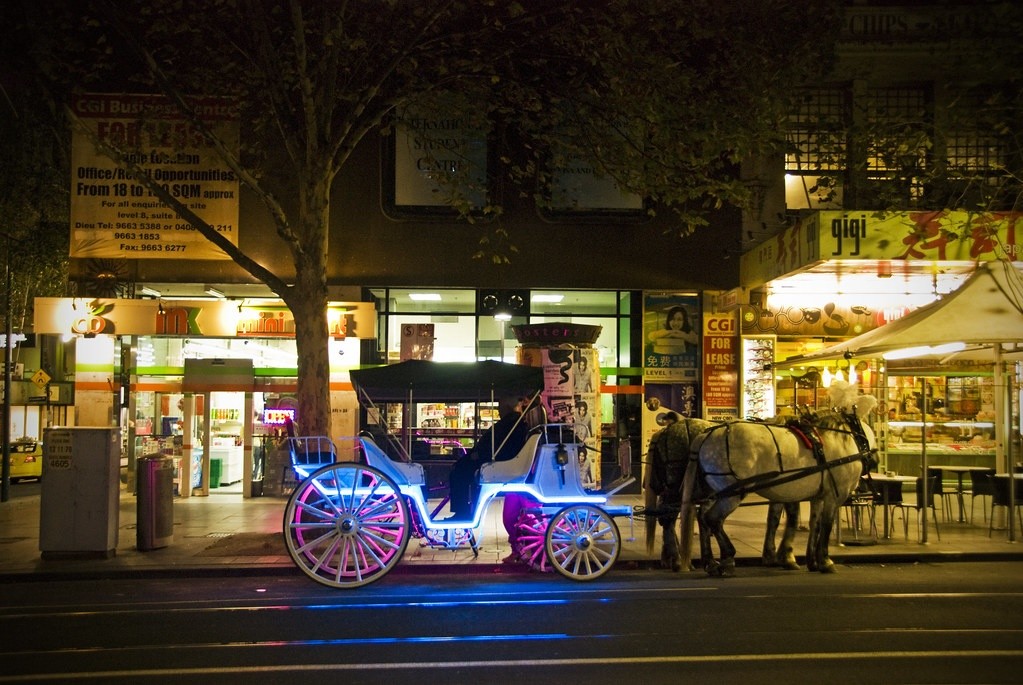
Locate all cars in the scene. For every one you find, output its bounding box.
[0,438,42,485]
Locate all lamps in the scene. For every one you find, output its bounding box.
[204,285,225,299]
[138,285,161,298]
[236,300,245,314]
[156,299,169,315]
[492,307,513,322]
[554,444,569,486]
[71,294,82,311]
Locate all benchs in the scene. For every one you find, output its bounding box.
[479,430,542,483]
[285,416,338,464]
[359,430,424,484]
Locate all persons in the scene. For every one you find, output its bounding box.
[444,398,528,520]
[648,307,699,345]
[254,411,261,480]
[889,409,905,443]
[574,356,592,393]
[579,448,595,485]
[662,412,678,426]
[576,402,594,440]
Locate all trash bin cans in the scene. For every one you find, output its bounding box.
[136,453,175,552]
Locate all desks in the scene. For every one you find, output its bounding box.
[994,472,1023,531]
[861,474,918,538]
[928,465,991,523]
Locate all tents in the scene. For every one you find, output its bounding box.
[763,259,1023,545]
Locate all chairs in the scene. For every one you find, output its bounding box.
[989,476,1023,540]
[836,501,880,539]
[525,403,588,447]
[871,478,906,537]
[957,469,997,525]
[920,466,968,524]
[889,476,941,544]
[852,491,881,530]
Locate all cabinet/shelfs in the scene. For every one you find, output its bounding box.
[945,376,982,418]
[476,406,501,429]
[889,420,996,492]
[135,434,182,496]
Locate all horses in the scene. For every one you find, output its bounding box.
[645,408,876,579]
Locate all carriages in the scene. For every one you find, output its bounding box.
[280,360,880,588]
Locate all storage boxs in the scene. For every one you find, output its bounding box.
[135,419,152,434]
[917,398,930,413]
[931,433,949,438]
[387,413,398,423]
[162,448,173,455]
[209,459,223,488]
[174,435,183,445]
[931,438,939,443]
[163,417,178,435]
[147,440,159,454]
[212,437,235,447]
[939,436,954,445]
